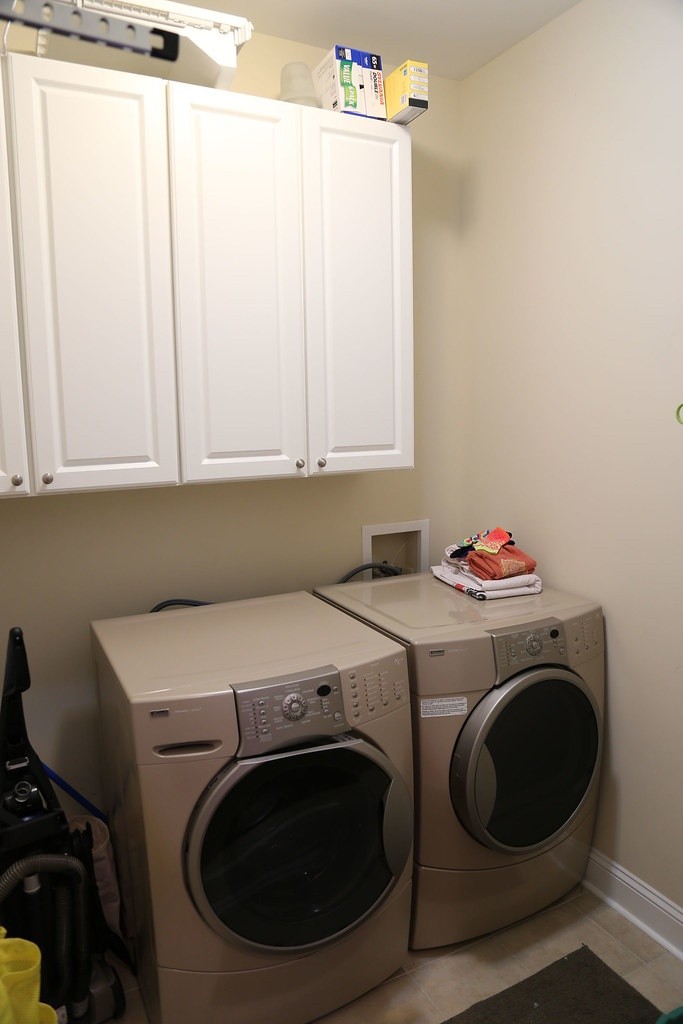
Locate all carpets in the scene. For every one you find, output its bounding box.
[443,944,665,1024]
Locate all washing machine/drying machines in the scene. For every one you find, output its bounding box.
[88,589,416,1024]
[311,569,606,952]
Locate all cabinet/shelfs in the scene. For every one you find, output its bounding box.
[169,78,416,487]
[0,46,182,502]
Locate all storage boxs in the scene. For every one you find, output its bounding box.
[359,51,399,118]
[313,44,368,116]
[385,60,430,124]
